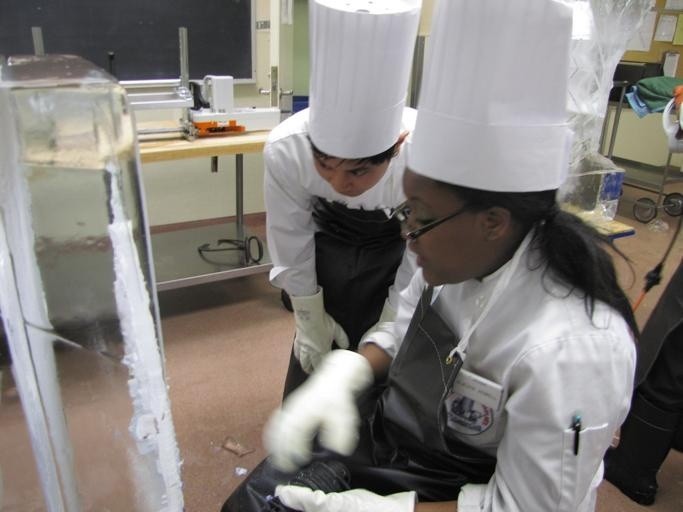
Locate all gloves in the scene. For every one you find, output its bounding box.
[288,286,350,376]
[261,349,375,472]
[275,484,417,512]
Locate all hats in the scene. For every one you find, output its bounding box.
[305,0,420,159]
[404,0,576,193]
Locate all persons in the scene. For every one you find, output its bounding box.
[219,164,640,510]
[593,256,682,507]
[258,105,429,459]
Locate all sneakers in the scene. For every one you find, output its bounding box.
[603,446,658,506]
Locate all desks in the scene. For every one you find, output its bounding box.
[21,122,273,326]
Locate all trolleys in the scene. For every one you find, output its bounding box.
[596,80,683,224]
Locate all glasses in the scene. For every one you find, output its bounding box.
[391,197,482,240]
